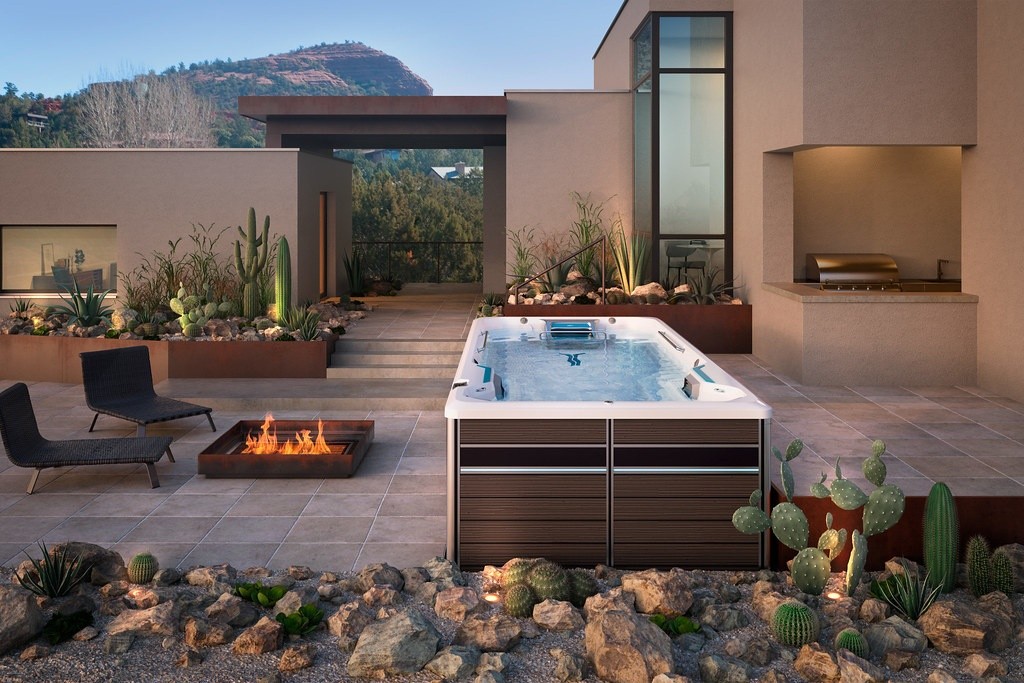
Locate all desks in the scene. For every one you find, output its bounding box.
[677,244,724,289]
[33,268,103,292]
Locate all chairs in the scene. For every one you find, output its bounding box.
[79,346,217,436]
[0,382,175,496]
[665,241,706,287]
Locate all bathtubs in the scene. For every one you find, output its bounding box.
[442,316,776,572]
